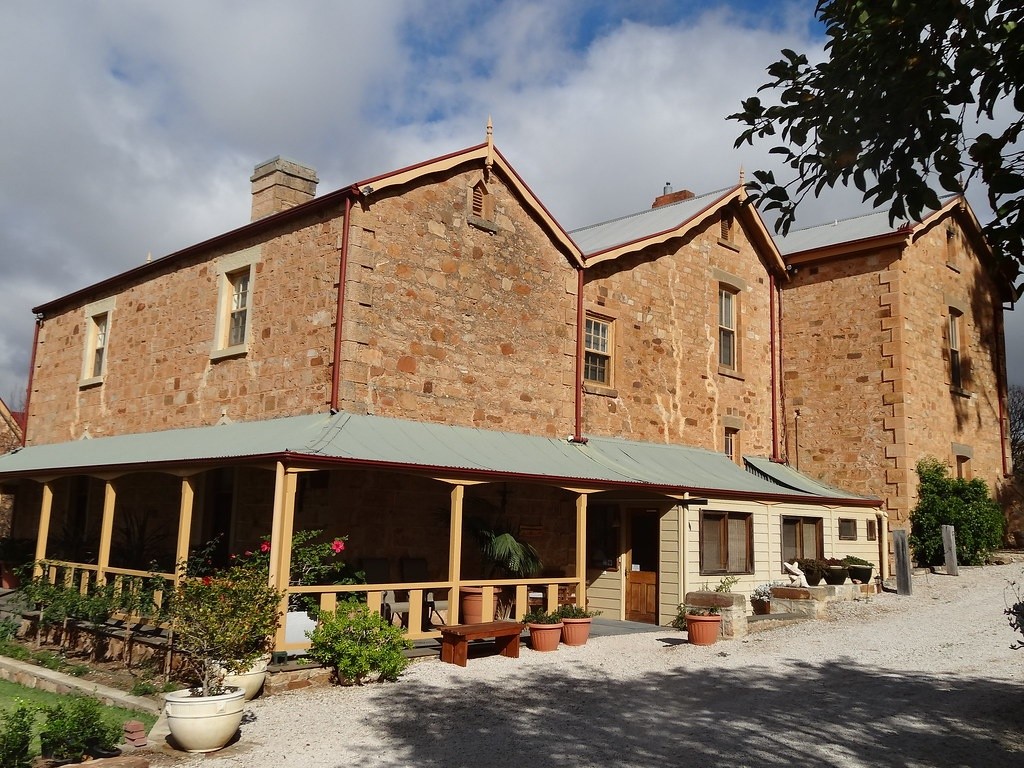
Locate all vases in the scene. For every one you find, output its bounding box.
[749,599,770,615]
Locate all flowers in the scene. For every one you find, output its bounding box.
[750,579,785,599]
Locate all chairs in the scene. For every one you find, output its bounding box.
[400,557,448,629]
[359,558,410,628]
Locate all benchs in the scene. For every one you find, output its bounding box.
[436,621,526,667]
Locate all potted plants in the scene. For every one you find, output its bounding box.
[817,557,853,585]
[0,540,26,588]
[558,605,604,646]
[459,519,544,625]
[521,608,565,652]
[684,606,722,646]
[156,593,263,753]
[846,555,876,584]
[182,566,285,699]
[297,595,416,685]
[784,557,824,586]
[0,685,121,768]
